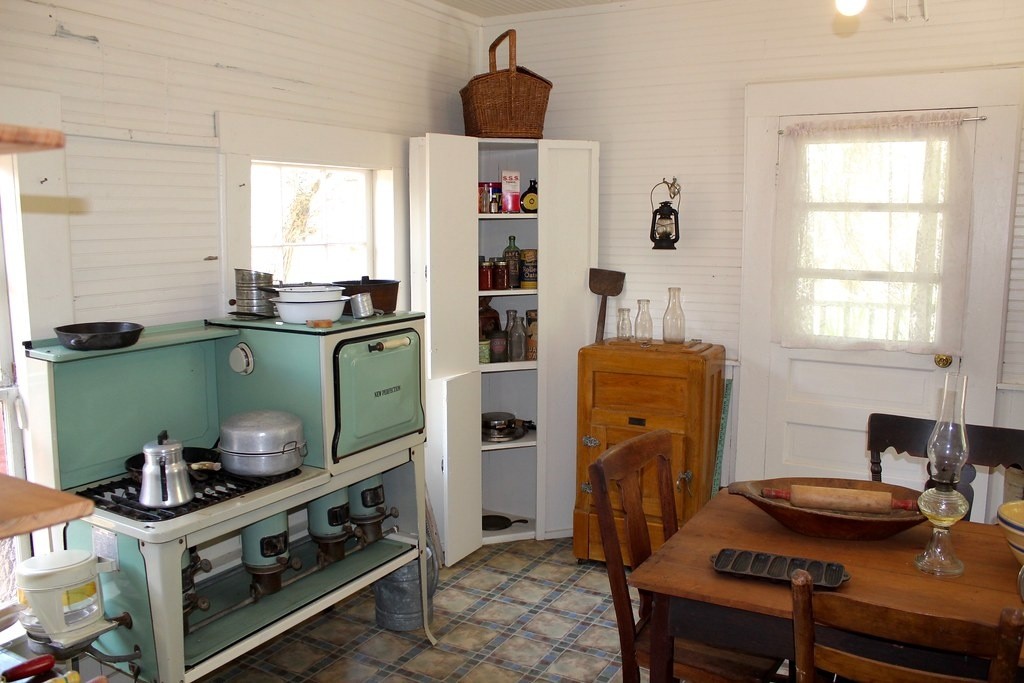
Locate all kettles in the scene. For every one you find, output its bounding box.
[139,430,194,508]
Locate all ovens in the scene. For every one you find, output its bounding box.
[203,311,427,477]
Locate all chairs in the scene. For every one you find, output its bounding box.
[865,411,1023,521]
[588,425,782,683]
[785,570,1024,683]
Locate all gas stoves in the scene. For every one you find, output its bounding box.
[74,464,330,544]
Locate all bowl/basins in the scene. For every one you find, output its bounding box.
[997,500,1024,567]
[333,280,400,315]
[268,285,351,325]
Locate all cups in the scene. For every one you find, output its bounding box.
[350,293,374,318]
[234,269,283,320]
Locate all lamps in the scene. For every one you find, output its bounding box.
[649,178,681,248]
[913,371,970,579]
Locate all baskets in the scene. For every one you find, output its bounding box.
[459,30,553,139]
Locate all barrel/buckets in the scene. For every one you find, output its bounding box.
[370,533,439,631]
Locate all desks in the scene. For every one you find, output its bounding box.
[626,481,1021,683]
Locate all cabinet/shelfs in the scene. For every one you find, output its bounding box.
[572,336,725,562]
[410,132,605,568]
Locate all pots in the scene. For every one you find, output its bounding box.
[54,322,145,351]
[124,446,222,485]
[15,549,117,638]
[482,412,517,439]
[216,410,309,478]
[481,515,529,531]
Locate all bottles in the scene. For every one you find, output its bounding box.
[520,179,538,213]
[663,287,685,344]
[617,308,631,340]
[505,310,526,361]
[481,184,489,212]
[635,299,653,342]
[489,195,499,213]
[479,261,509,289]
[503,236,521,289]
